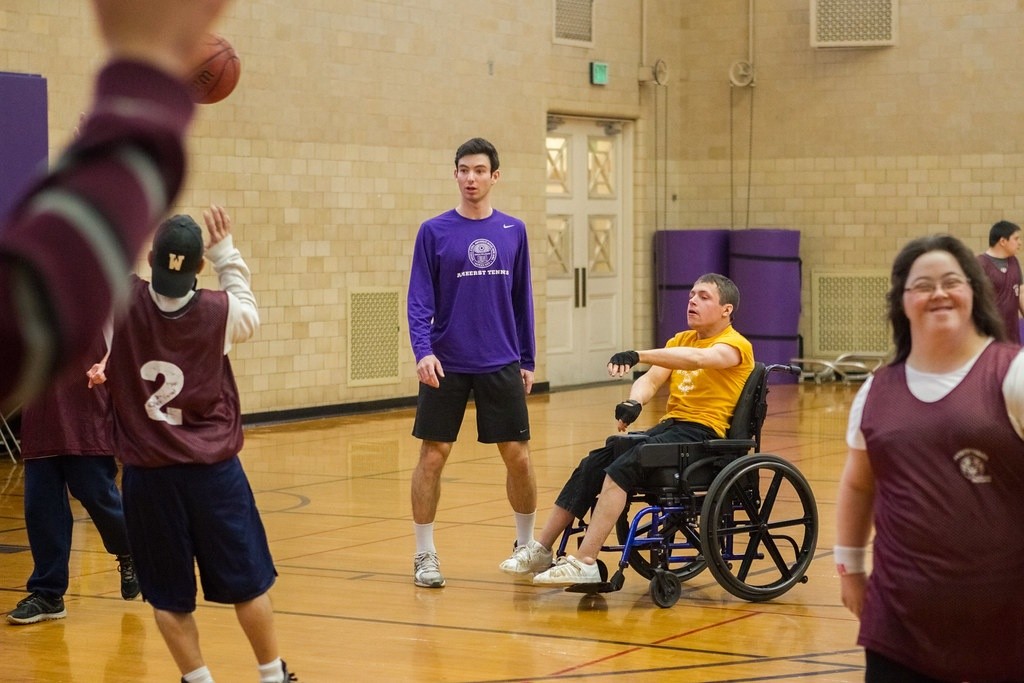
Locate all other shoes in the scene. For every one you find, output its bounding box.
[275,658,298,683]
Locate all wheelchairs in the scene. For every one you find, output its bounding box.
[549,361,818,611]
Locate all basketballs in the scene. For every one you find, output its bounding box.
[179,30,243,106]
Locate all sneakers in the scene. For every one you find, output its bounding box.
[499,541,553,576]
[513,540,555,576]
[115,554,141,601]
[533,555,602,586]
[412,553,446,587]
[6,593,67,624]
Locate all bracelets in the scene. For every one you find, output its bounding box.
[833,546,866,575]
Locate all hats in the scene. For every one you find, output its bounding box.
[152,215,203,297]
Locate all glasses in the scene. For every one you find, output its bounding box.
[903,277,971,296]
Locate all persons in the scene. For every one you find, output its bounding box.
[0,0,297,683]
[500,274,755,589]
[834,221,1024,683]
[410,140,537,589]
